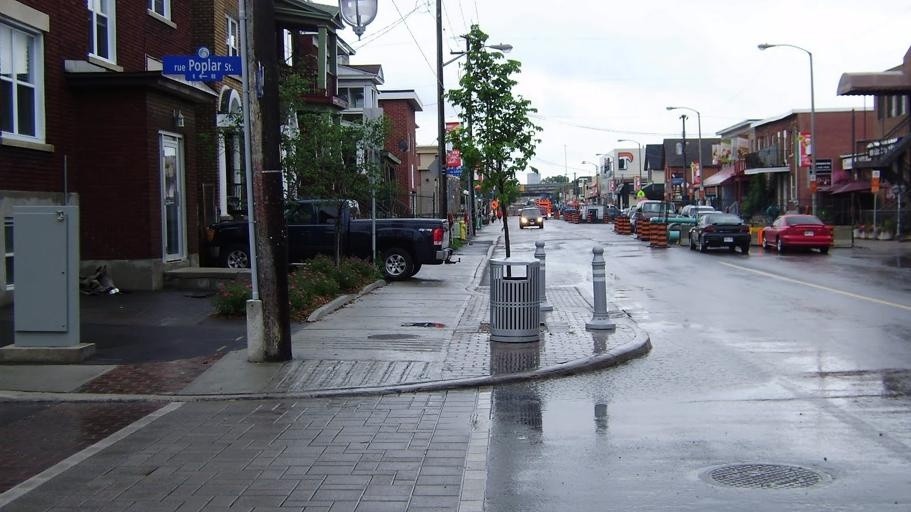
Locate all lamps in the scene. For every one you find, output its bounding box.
[172,109,185,128]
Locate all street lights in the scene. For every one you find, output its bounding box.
[758,44,817,215]
[238,0,378,362]
[596,154,615,205]
[582,161,598,206]
[667,107,704,206]
[618,139,642,191]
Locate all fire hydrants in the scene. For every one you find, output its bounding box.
[459,221,466,239]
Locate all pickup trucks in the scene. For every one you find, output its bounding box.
[198,199,453,281]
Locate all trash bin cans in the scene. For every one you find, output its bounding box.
[489,257,541,343]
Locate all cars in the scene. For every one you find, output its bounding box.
[606,199,680,234]
[688,213,751,254]
[680,205,722,218]
[519,207,543,229]
[762,214,834,253]
